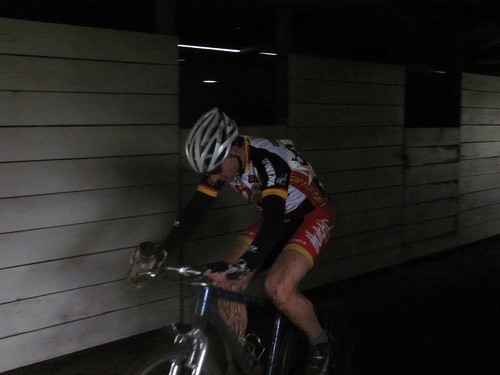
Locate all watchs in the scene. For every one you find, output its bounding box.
[233,259,248,272]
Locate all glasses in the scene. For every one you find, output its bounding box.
[194,166,222,180]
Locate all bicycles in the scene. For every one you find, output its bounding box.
[126,265,350,374]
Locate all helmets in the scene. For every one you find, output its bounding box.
[185,107,239,177]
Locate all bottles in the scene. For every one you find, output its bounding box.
[128,246,156,288]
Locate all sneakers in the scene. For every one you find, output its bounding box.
[303,330,335,375]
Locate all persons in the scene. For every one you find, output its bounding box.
[132,107,339,375]
